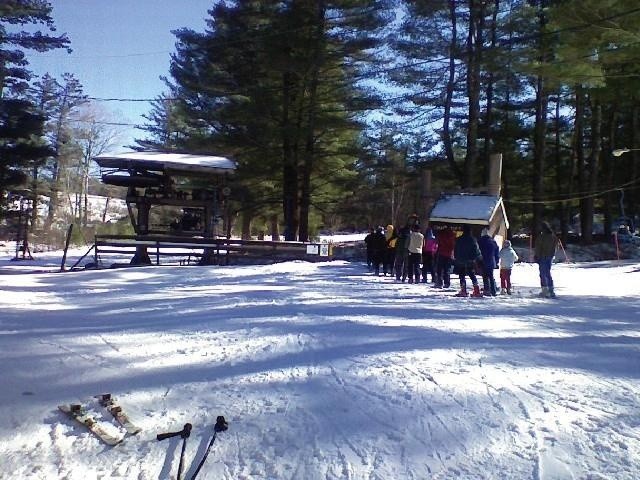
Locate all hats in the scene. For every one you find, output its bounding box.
[375,223,512,247]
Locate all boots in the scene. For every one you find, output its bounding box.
[367,268,556,298]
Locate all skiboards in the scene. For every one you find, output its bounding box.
[59,393,144,445]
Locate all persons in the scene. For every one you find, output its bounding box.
[363,213,519,297]
[534,218,560,300]
